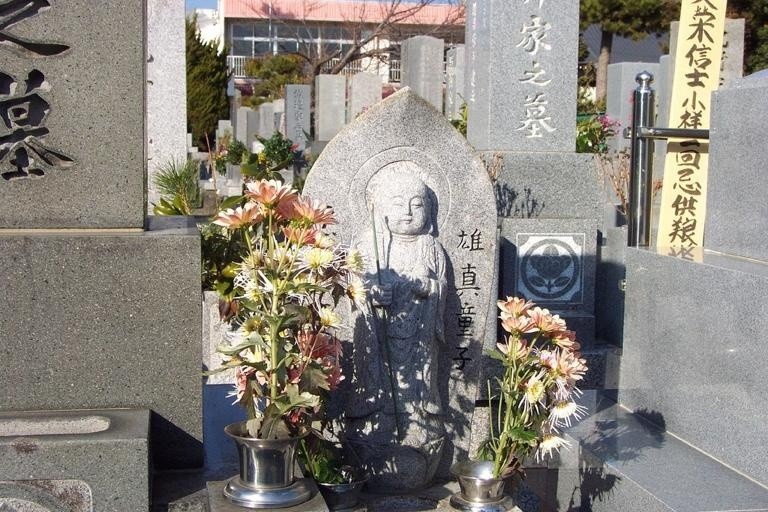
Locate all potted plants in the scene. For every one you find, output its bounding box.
[297,437,372,510]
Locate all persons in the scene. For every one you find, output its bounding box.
[335,165,444,490]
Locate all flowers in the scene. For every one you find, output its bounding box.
[485,297,589,478]
[211,179,372,434]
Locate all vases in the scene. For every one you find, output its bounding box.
[223,419,313,508]
[449,459,516,512]
